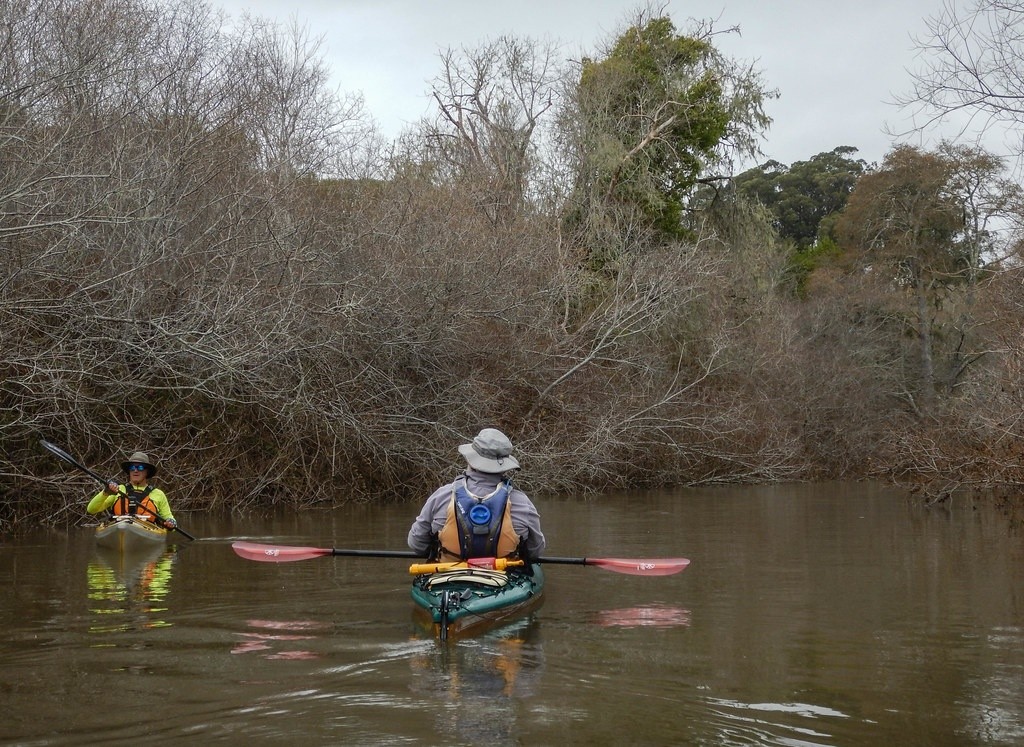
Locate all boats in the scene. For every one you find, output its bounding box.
[95,513,168,553]
[410,560,545,641]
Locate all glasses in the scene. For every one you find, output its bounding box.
[128,464,148,471]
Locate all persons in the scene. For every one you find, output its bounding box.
[407,427,546,564]
[87,452,177,531]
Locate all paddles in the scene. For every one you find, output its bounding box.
[230,540,692,579]
[38,438,196,541]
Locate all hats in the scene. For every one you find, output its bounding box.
[120,452,157,479]
[458,428,521,474]
